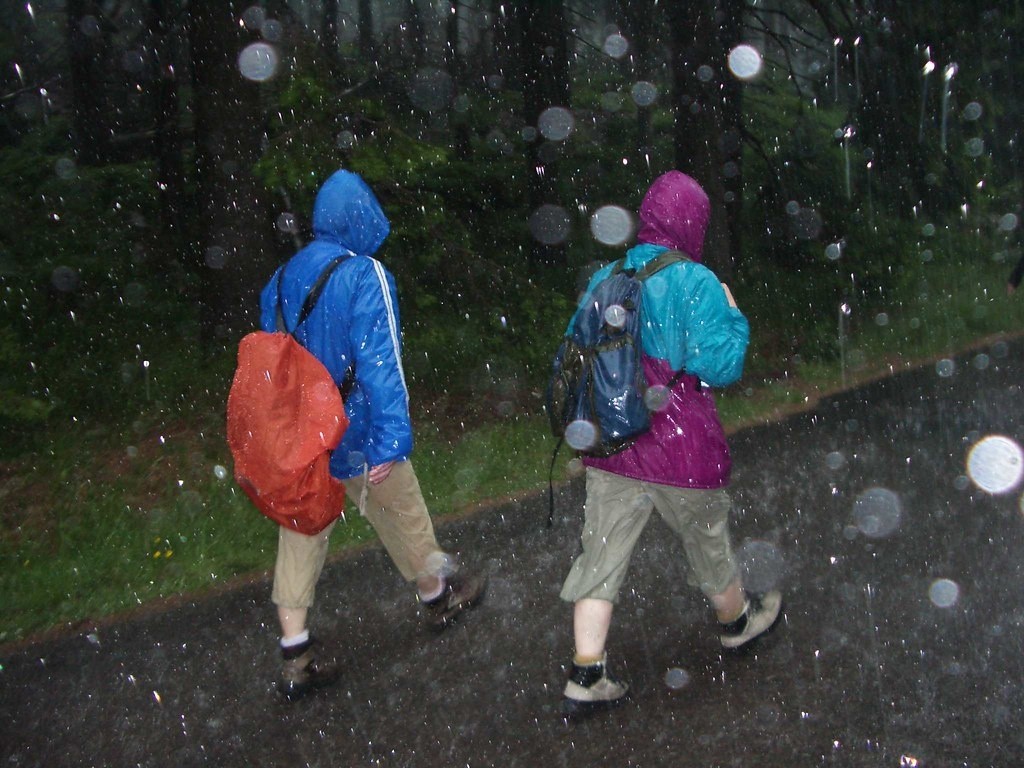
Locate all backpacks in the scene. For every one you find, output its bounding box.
[226,254,355,534]
[548,249,684,458]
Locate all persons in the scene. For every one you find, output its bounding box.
[559,171,782,702]
[260,171,487,684]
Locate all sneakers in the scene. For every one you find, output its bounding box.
[272,645,344,705]
[558,652,629,723]
[415,568,487,635]
[717,588,785,652]
